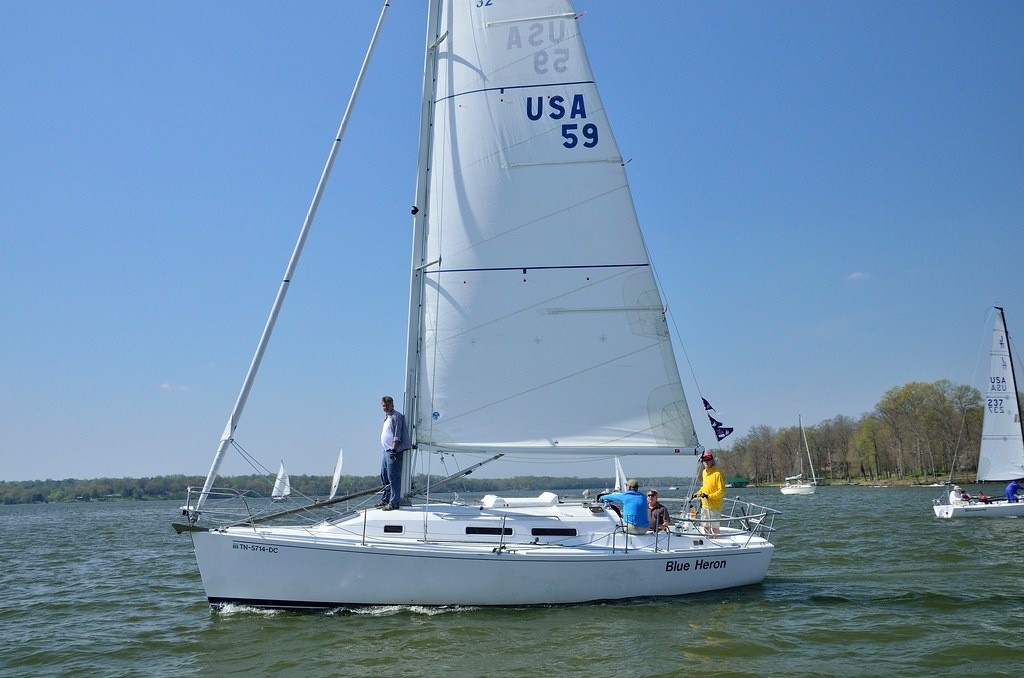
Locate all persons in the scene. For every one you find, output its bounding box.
[692,452,724,538]
[959,490,971,501]
[1005,480,1024,503]
[785,481,791,487]
[598,479,652,534]
[372,396,411,511]
[646,488,670,534]
[797,480,803,485]
[949,485,967,505]
[978,491,988,502]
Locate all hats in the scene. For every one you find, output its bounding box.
[624,479,638,490]
[703,453,714,459]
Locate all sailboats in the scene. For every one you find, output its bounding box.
[924,307,1024,521]
[269,460,292,501]
[170,0,781,616]
[781,414,818,495]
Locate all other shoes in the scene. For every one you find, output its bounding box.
[382,503,399,510]
[373,500,388,507]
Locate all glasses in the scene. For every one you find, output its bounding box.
[703,458,711,461]
[647,494,657,497]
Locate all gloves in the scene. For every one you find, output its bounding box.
[702,493,708,499]
[693,494,697,497]
